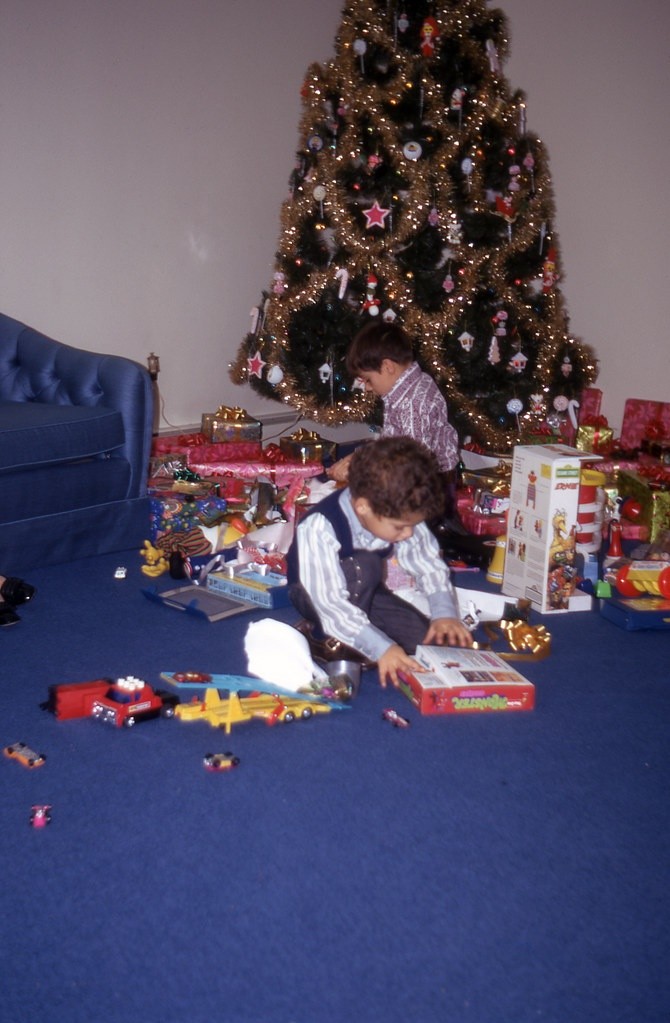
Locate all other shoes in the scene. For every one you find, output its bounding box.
[303,617,378,671]
[0,602,21,627]
[0,575,35,606]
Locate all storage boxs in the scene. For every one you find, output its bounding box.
[396,645,533,715]
[139,387,670,631]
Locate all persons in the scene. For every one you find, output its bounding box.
[322,320,462,485]
[284,436,475,700]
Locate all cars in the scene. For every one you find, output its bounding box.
[203,751,240,772]
[92,676,180,728]
[382,707,410,728]
[173,670,211,684]
[4,743,47,769]
[29,803,53,828]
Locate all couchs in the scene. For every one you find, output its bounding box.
[0,311,152,577]
